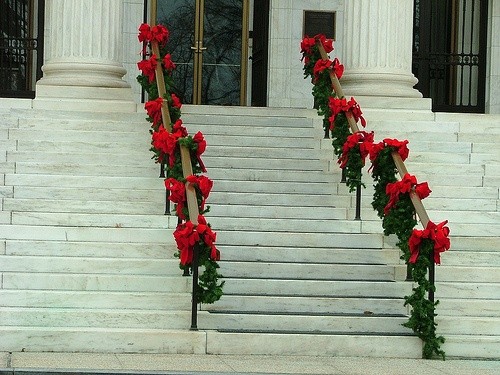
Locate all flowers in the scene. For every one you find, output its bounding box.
[312,58,345,84]
[404,219,451,266]
[172,214,221,268]
[151,119,208,173]
[164,174,214,221]
[143,93,183,127]
[327,96,367,131]
[367,138,411,173]
[136,53,176,85]
[300,33,335,65]
[381,173,432,215]
[136,23,170,46]
[336,130,375,170]
